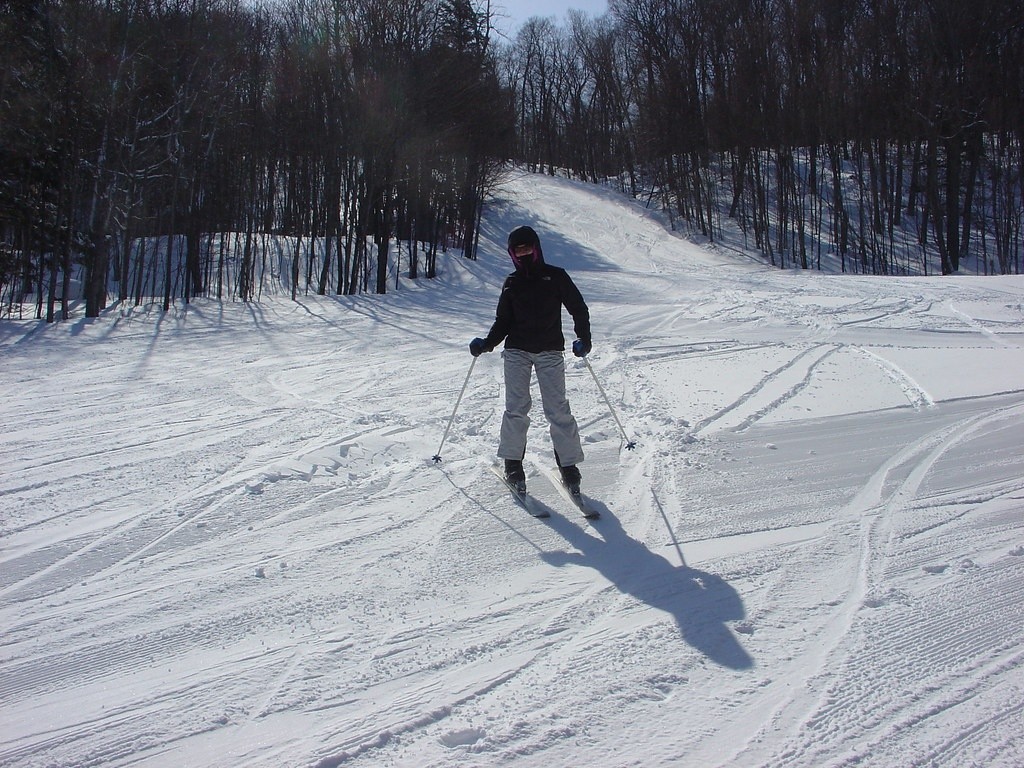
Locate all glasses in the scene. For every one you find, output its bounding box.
[515,245,532,254]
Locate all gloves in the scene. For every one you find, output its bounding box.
[573,339,591,357]
[470,338,494,357]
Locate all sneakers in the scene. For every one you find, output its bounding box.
[559,465,582,487]
[505,458,526,493]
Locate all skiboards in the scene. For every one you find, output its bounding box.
[490,460,601,519]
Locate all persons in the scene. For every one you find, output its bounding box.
[469,225,592,497]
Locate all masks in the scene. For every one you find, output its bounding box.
[516,250,535,269]
